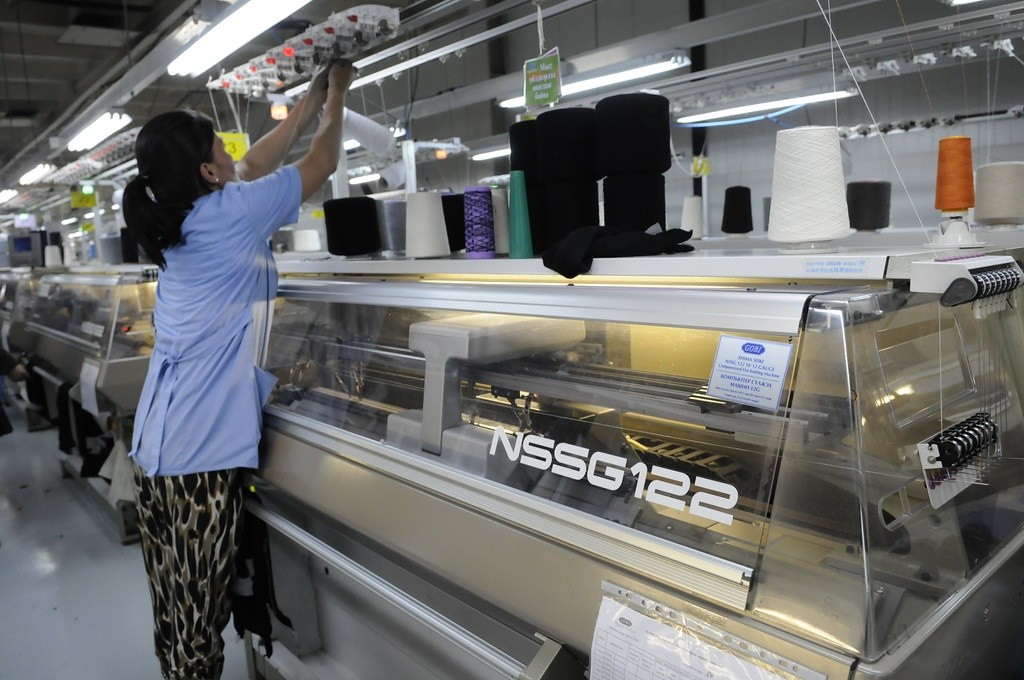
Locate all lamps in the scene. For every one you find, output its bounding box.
[494,47,692,109]
[67,0,132,151]
[0,0,57,185]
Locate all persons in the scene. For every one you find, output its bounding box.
[122,56,361,680]
[0,343,32,437]
